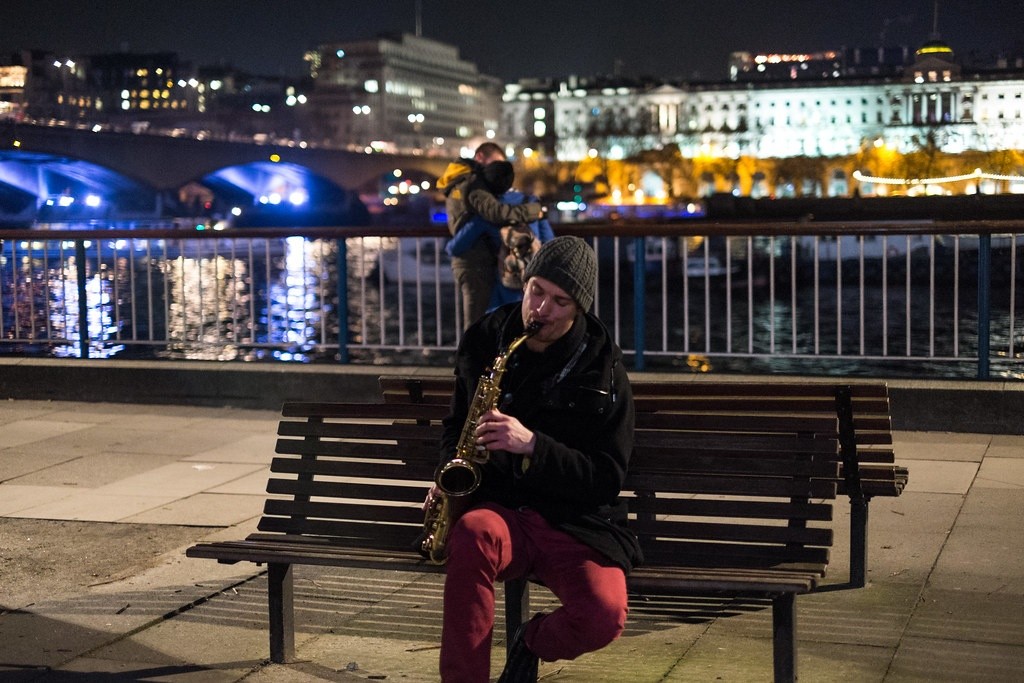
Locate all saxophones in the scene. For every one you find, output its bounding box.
[417,320,544,566]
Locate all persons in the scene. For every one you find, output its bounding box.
[423,236,633,683]
[436,143,555,332]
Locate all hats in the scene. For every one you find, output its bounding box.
[485,161,514,192]
[524,235,596,314]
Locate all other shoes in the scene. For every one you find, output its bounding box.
[499,622,539,683]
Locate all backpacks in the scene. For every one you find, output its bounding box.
[488,221,542,289]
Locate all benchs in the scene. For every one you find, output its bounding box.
[378,373,911,587]
[186,401,840,683]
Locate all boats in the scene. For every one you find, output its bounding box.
[376,235,1024,285]
